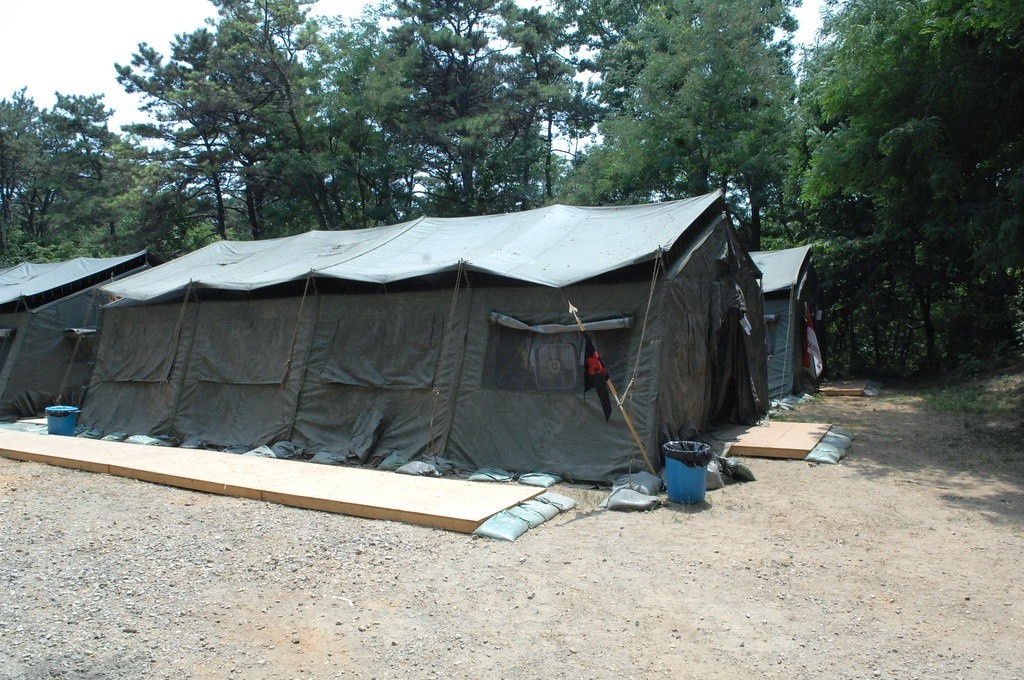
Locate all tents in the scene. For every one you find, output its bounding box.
[0,245,156,426]
[748,244,823,400]
[75,191,769,486]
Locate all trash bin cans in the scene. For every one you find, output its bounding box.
[45,406,79,436]
[661,440,711,505]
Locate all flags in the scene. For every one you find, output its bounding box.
[581,330,613,422]
[803,305,823,380]
